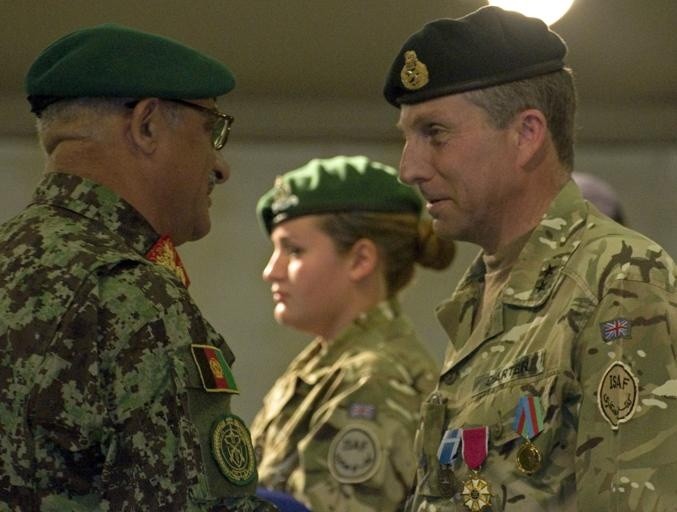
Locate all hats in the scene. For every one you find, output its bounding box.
[254,154,426,237]
[23,21,238,99]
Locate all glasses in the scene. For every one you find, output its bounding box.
[124,97,235,151]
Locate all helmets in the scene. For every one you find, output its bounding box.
[381,5,571,110]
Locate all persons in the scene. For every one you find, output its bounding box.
[570,172,625,228]
[248,154,455,512]
[0,24,304,511]
[382,8,677,512]
